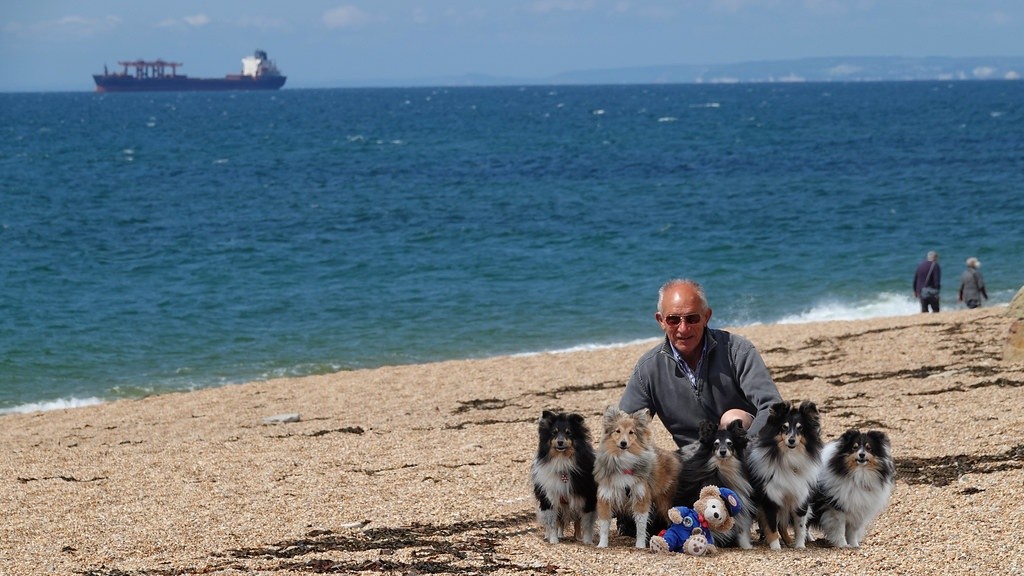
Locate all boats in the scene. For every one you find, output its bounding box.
[90,50,287,93]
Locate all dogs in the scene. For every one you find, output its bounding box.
[679,420,755,549]
[532,410,598,545]
[744,402,824,550]
[594,406,684,548]
[806,428,896,548]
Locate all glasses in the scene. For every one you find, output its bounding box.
[666,314,701,326]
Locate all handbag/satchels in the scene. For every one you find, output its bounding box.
[921,287,937,299]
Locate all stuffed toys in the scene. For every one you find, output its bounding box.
[649,484,742,557]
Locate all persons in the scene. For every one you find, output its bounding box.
[959,257,989,310]
[913,251,942,312]
[616,278,785,456]
